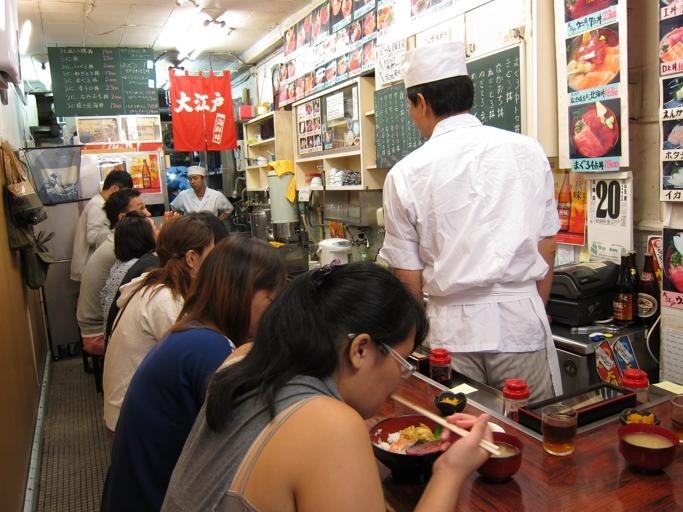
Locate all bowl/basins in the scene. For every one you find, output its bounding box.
[475,431,522,480]
[571,106,617,159]
[371,415,457,483]
[435,391,465,414]
[617,422,680,466]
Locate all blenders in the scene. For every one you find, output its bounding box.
[317,238,352,267]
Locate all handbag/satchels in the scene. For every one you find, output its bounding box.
[3,140,35,249]
[19,231,56,290]
[2,140,48,226]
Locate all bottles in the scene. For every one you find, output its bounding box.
[619,367,650,406]
[141,160,151,188]
[327,202,352,218]
[428,348,452,384]
[612,250,659,323]
[503,379,530,424]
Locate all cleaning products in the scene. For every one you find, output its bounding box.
[358,244,368,261]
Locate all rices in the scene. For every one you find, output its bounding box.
[372,429,410,455]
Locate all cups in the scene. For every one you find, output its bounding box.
[667,396,683,428]
[541,405,577,457]
[620,407,661,427]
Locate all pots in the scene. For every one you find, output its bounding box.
[271,221,300,241]
[252,210,270,239]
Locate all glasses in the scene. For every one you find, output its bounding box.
[347,333,417,380]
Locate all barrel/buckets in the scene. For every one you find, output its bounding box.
[250,211,267,239]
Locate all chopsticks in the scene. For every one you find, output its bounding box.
[388,395,500,455]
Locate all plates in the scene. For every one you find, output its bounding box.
[564,29,621,91]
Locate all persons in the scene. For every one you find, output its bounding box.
[97,232,288,511]
[70,164,235,443]
[377,39,564,406]
[155,260,495,512]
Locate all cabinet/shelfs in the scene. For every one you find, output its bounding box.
[291,76,386,192]
[242,111,292,192]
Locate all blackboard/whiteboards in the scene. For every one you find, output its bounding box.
[48,47,160,116]
[374,41,526,168]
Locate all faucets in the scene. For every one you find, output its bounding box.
[363,232,371,248]
[232,177,245,199]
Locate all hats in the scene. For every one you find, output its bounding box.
[402,40,469,89]
[187,166,206,178]
